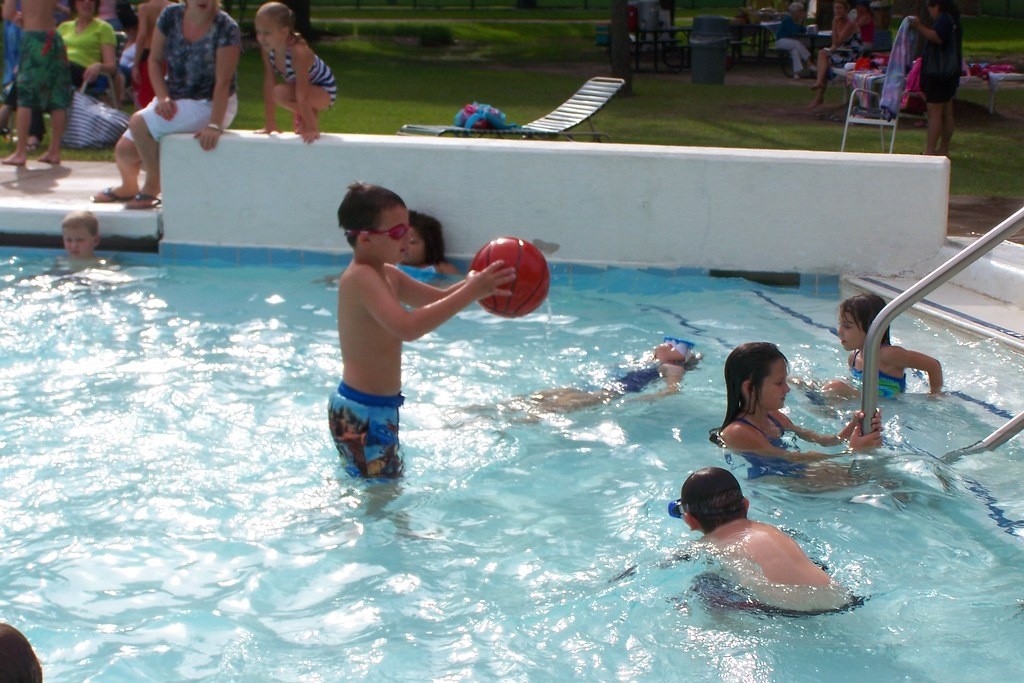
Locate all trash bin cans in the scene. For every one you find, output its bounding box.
[688,16,733,85]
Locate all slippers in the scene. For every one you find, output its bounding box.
[125,192,162,209]
[93,188,141,203]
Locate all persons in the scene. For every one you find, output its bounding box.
[0,622,44,683]
[806,0,875,114]
[61,210,100,259]
[254,0,337,143]
[0,0,179,167]
[90,0,243,209]
[908,0,964,160]
[721,341,884,451]
[825,295,944,399]
[326,182,516,475]
[775,1,818,81]
[398,207,459,276]
[680,467,832,588]
[734,6,756,24]
[655,340,690,362]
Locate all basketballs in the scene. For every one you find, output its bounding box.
[471,239,550,321]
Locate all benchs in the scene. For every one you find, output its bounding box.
[595,25,691,68]
[767,43,830,75]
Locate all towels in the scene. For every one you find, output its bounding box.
[878,14,921,124]
[845,69,888,113]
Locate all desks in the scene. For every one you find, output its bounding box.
[731,19,783,65]
[829,65,1023,114]
[634,25,692,71]
[800,29,834,78]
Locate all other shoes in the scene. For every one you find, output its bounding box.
[807,64,817,71]
[794,74,800,80]
[25,141,40,152]
[0,126,10,136]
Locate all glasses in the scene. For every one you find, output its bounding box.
[664,336,694,362]
[667,499,686,519]
[345,224,414,241]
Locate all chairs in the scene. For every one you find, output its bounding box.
[871,30,895,51]
[395,76,627,143]
[841,89,925,153]
[0,32,138,141]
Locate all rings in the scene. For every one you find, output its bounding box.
[166,110,169,113]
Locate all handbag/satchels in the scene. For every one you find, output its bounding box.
[58,91,131,149]
[739,6,761,24]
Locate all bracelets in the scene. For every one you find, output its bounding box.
[207,123,221,130]
